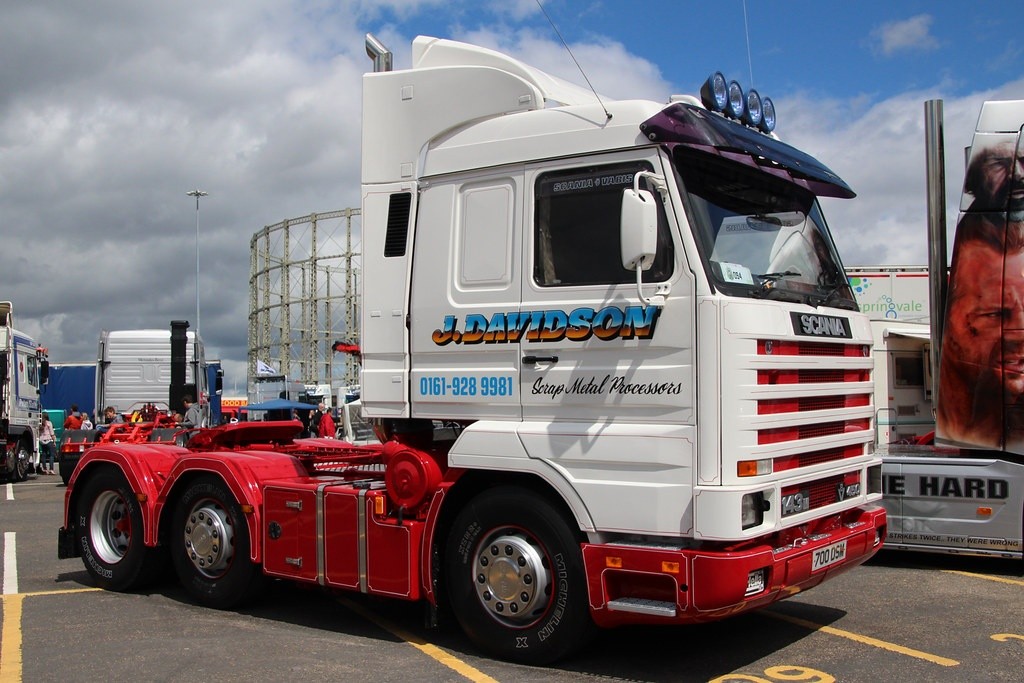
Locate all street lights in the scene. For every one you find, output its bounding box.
[186,190,210,334]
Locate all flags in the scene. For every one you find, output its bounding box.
[257,360,276,374]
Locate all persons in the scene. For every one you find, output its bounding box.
[222,402,336,439]
[940,138,1024,454]
[39,394,204,474]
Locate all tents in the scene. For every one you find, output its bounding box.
[237,398,318,421]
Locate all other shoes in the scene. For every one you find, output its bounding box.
[49,470,55,475]
[43,469,48,475]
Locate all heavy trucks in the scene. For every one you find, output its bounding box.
[59,31,888,668]
[59,328,224,486]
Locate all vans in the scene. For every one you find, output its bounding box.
[298,383,361,419]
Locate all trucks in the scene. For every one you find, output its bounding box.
[0,300,49,484]
[834,100,1024,559]
